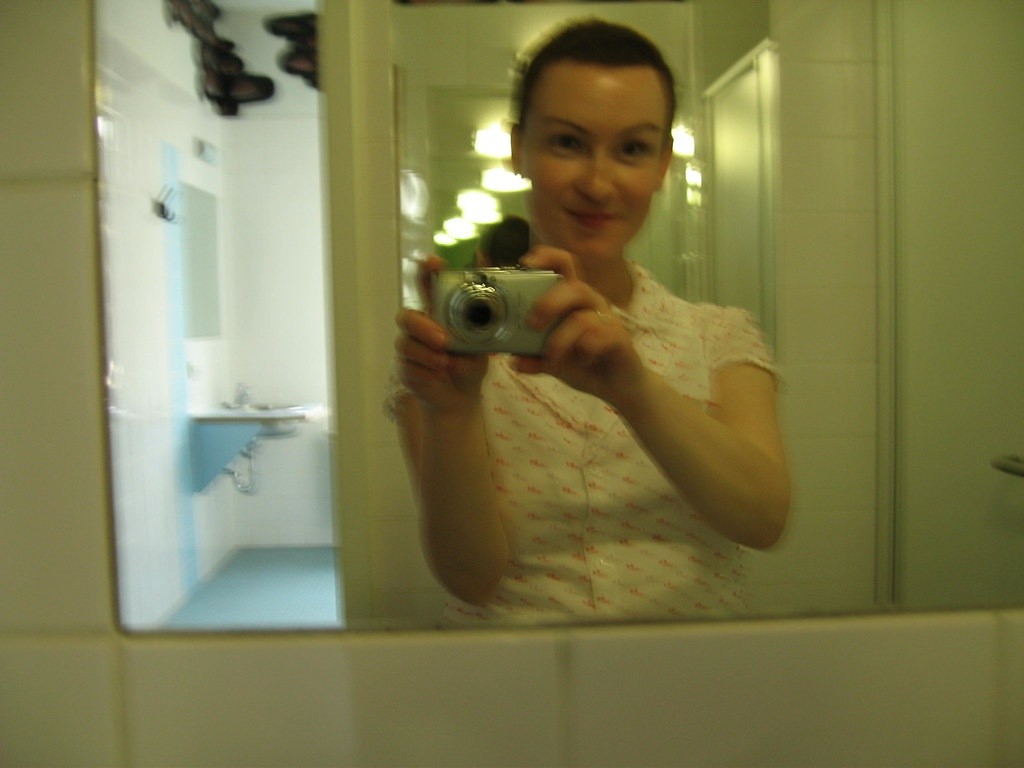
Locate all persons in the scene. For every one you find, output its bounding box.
[383,14,792,632]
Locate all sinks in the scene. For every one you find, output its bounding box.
[190,398,318,439]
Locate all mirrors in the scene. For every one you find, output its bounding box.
[90,0,1024,635]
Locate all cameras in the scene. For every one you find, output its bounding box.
[425,266,567,356]
